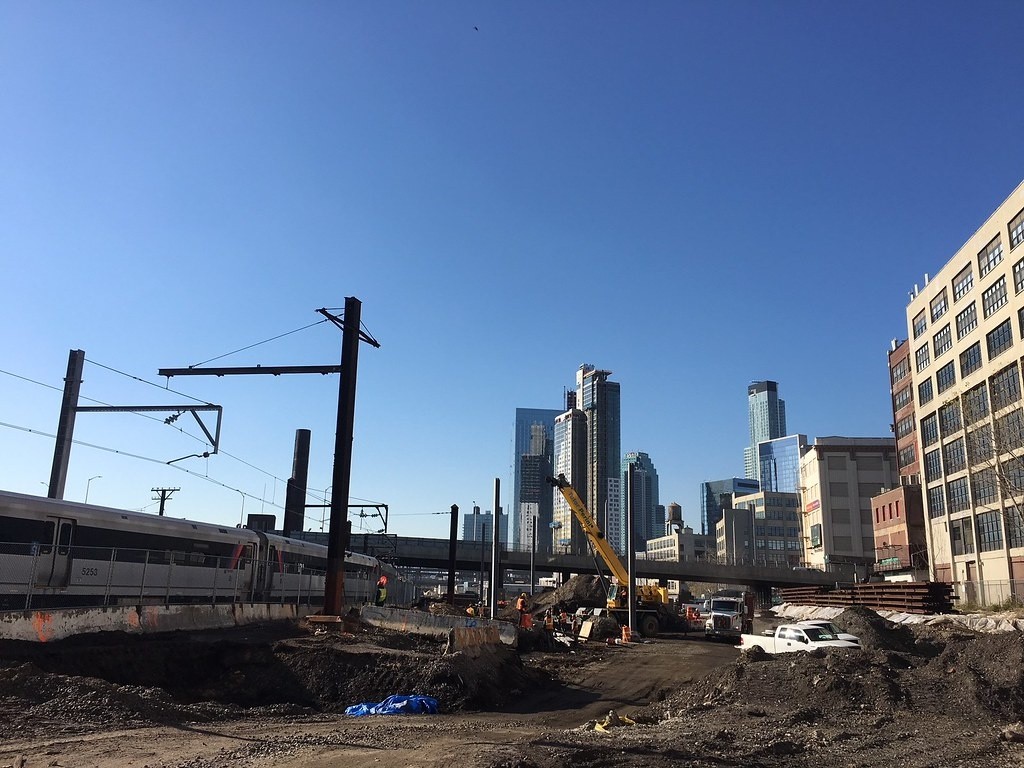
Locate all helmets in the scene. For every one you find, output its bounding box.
[377,581,383,585]
[521,593,526,597]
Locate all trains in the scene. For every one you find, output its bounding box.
[2,485,417,645]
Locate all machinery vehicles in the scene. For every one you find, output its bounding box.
[541,471,677,637]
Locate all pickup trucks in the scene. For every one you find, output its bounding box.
[734,625,863,654]
[762,620,860,645]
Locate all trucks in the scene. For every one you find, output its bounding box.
[679,603,711,631]
[455,583,480,594]
[425,584,448,597]
[705,591,755,642]
[497,600,511,608]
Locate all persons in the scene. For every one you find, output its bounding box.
[693,608,700,618]
[543,609,583,648]
[375,581,386,607]
[516,593,529,628]
[466,601,483,616]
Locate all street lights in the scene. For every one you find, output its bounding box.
[471,499,480,541]
[602,498,608,541]
[233,487,246,529]
[84,474,102,504]
